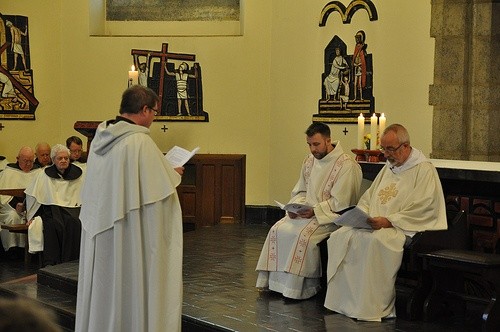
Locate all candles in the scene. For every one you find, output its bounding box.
[129,65,139,87]
[370,113,378,150]
[357,113,365,150]
[378,112,386,150]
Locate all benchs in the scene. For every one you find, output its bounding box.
[1,223,30,270]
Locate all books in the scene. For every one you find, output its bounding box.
[329,205,373,229]
[165,145,200,167]
[275,200,315,215]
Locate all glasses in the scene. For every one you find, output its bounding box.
[148,106,159,116]
[379,142,405,156]
[69,148,83,153]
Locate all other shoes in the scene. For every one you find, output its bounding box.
[268,291,283,299]
[284,298,309,304]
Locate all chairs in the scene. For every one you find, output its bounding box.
[316,205,500,332]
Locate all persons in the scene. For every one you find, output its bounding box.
[254,123,363,303]
[74,85,185,332]
[0,136,88,268]
[323,123,448,322]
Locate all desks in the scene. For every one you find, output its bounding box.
[0,188,25,197]
[358,158,500,204]
